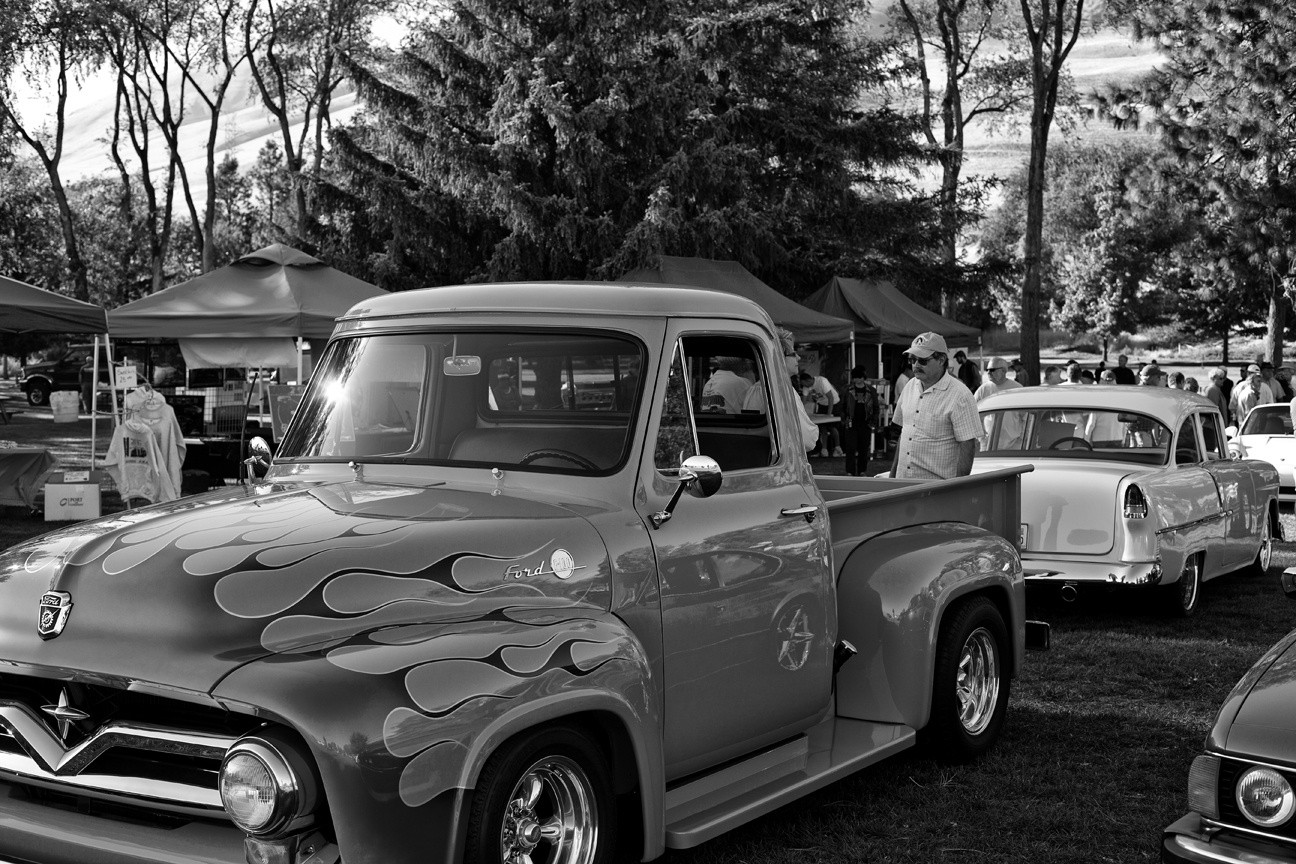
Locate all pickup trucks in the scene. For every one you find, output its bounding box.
[0,275,1055,864]
[16,343,184,407]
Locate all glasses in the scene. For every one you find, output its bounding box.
[1279,368,1286,372]
[784,350,799,360]
[1215,377,1224,381]
[985,366,1004,373]
[907,356,938,366]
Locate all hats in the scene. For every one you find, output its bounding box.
[1141,364,1166,376]
[1074,369,1094,380]
[1259,362,1275,372]
[902,332,947,359]
[1247,364,1261,373]
[1009,359,1025,369]
[1279,362,1293,369]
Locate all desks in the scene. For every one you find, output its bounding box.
[0,448,59,512]
[808,412,841,424]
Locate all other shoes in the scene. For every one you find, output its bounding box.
[833,447,843,457]
[860,472,868,477]
[813,448,829,457]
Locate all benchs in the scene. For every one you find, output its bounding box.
[448,426,769,476]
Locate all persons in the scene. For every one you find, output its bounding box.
[610,360,640,410]
[1204,363,1296,440]
[974,357,1026,451]
[1111,355,1136,385]
[894,357,915,408]
[700,357,752,414]
[953,351,981,395]
[1009,359,1028,387]
[1136,360,1199,443]
[840,365,879,476]
[1118,364,1166,448]
[1041,361,1105,438]
[799,373,845,457]
[79,356,94,414]
[1083,369,1125,448]
[888,332,983,479]
[742,330,820,452]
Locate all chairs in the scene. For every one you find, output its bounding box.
[1262,417,1285,432]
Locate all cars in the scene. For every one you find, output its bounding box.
[875,378,1280,613]
[1218,398,1295,512]
[1154,564,1295,864]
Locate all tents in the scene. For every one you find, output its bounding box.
[0,276,121,427]
[487,255,855,409]
[801,276,983,461]
[92,242,391,469]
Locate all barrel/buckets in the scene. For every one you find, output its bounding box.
[50,390,79,423]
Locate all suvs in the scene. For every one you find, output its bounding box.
[522,356,638,412]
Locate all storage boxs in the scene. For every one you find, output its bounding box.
[164,395,205,434]
